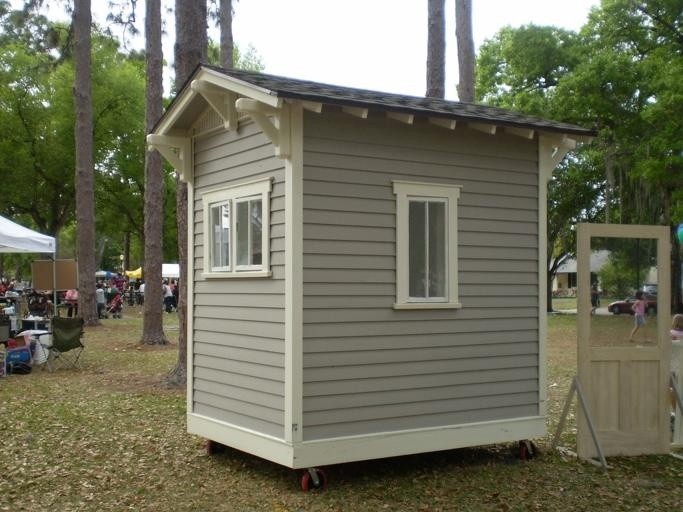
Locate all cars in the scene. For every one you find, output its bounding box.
[607,295,657,316]
[635,282,657,297]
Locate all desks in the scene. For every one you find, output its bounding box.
[21,317,50,330]
[16,329,49,364]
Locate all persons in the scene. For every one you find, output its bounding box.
[669,314,683,419]
[0,274,79,319]
[95,270,145,319]
[591,280,601,315]
[162,277,179,314]
[629,291,652,343]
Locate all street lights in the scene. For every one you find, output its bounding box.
[119,253,123,275]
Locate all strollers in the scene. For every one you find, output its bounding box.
[26,286,59,319]
[101,294,126,318]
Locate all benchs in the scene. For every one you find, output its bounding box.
[57,298,78,317]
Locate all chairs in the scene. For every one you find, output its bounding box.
[37,316,85,373]
